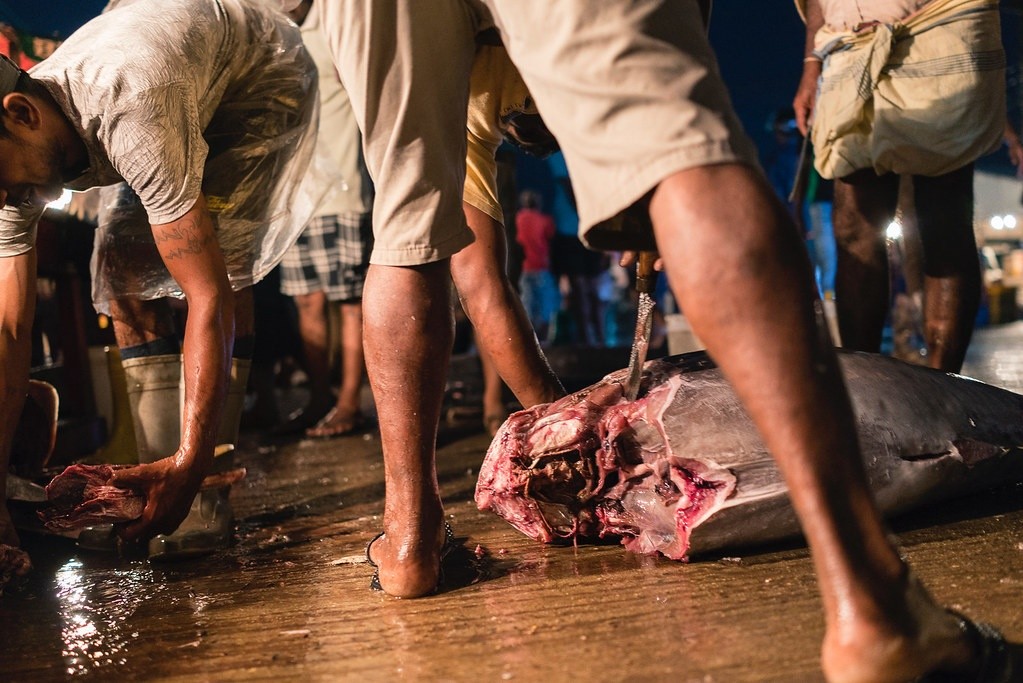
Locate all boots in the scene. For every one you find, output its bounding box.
[148,359,254,558]
[75,352,182,549]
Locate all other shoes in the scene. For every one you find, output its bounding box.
[484,406,509,443]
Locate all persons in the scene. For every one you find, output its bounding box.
[2,0,317,556]
[792,0,1023,372]
[276,0,375,438]
[324,1,1022,682]
[514,191,558,329]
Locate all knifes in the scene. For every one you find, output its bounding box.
[618,251,660,402]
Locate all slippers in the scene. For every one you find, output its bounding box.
[305,410,368,439]
[367,521,454,599]
[921,606,1014,681]
